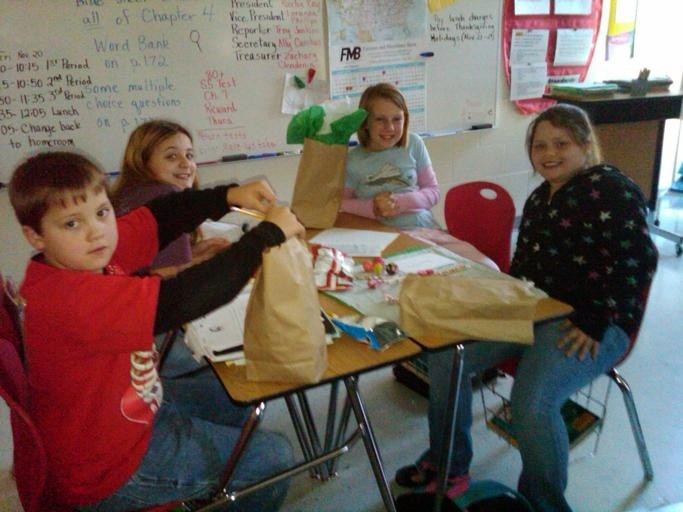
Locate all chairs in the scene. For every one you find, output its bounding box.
[3,276,27,351]
[2,338,192,510]
[390,181,518,401]
[481,232,653,484]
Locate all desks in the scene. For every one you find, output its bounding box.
[299,208,577,508]
[155,279,424,510]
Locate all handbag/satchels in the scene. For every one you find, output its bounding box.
[399,273,536,348]
[291,137,347,229]
[244,234,327,384]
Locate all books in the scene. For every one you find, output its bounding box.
[550,82,618,101]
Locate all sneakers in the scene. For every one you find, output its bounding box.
[396,460,469,486]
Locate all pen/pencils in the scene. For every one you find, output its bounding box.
[105,172,119,175]
[349,141,357,146]
[248,156,263,159]
[262,153,283,157]
[230,206,265,220]
[223,155,247,161]
[196,161,216,165]
[472,124,492,129]
[0,0,503,188]
[631,68,650,96]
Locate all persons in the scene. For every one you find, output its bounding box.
[338,82,442,232]
[397,103,660,512]
[7,150,309,512]
[111,120,230,278]
